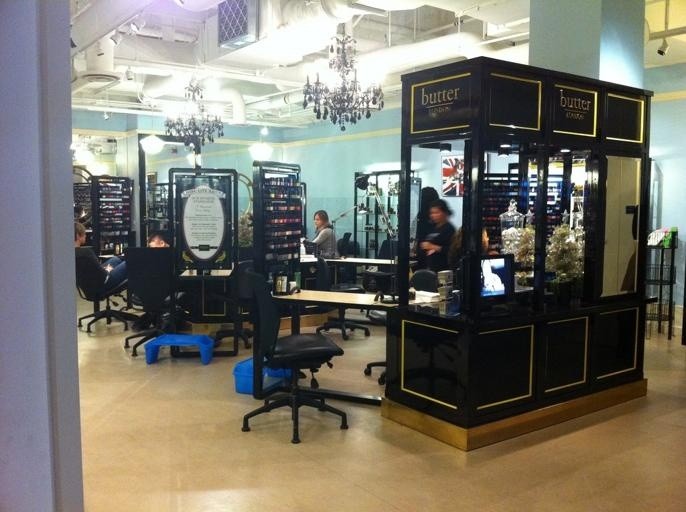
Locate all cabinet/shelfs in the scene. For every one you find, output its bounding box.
[380,56,654,453]
[90,174,134,258]
[252,161,302,330]
[73,182,91,218]
[350,168,423,261]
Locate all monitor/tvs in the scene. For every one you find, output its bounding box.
[478,253,514,299]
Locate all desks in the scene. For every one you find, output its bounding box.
[325,258,419,267]
[272,290,440,407]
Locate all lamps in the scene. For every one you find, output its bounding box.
[303,24,384,132]
[164,74,224,156]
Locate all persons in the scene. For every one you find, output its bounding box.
[416,186,439,269]
[148,229,173,248]
[311,210,336,271]
[73,206,92,229]
[74,222,127,297]
[420,199,456,273]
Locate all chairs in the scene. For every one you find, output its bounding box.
[364,268,439,385]
[216,260,254,348]
[328,232,352,265]
[76,255,141,332]
[241,269,349,444]
[122,246,185,357]
[314,263,370,341]
